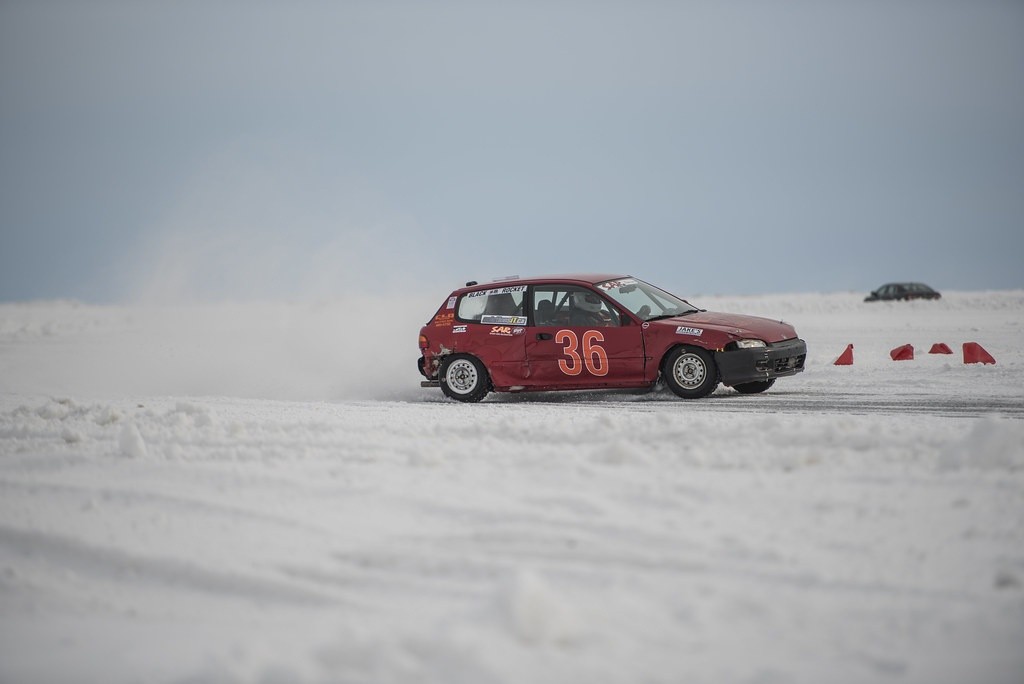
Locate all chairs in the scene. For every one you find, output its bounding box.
[472,293,522,321]
[538,299,553,322]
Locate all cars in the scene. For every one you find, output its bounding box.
[862,280,942,302]
[417,271,807,403]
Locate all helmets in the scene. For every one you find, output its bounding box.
[573,292,602,313]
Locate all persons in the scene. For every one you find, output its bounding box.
[568,291,620,326]
